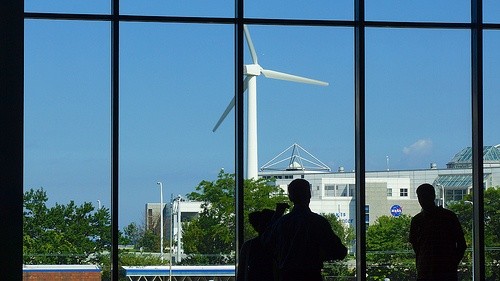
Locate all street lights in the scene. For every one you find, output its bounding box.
[97,200,100,210]
[157,182,164,265]
[437,184,445,209]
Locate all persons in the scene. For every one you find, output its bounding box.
[236,206,283,281]
[265,177,349,281]
[408,183,467,281]
